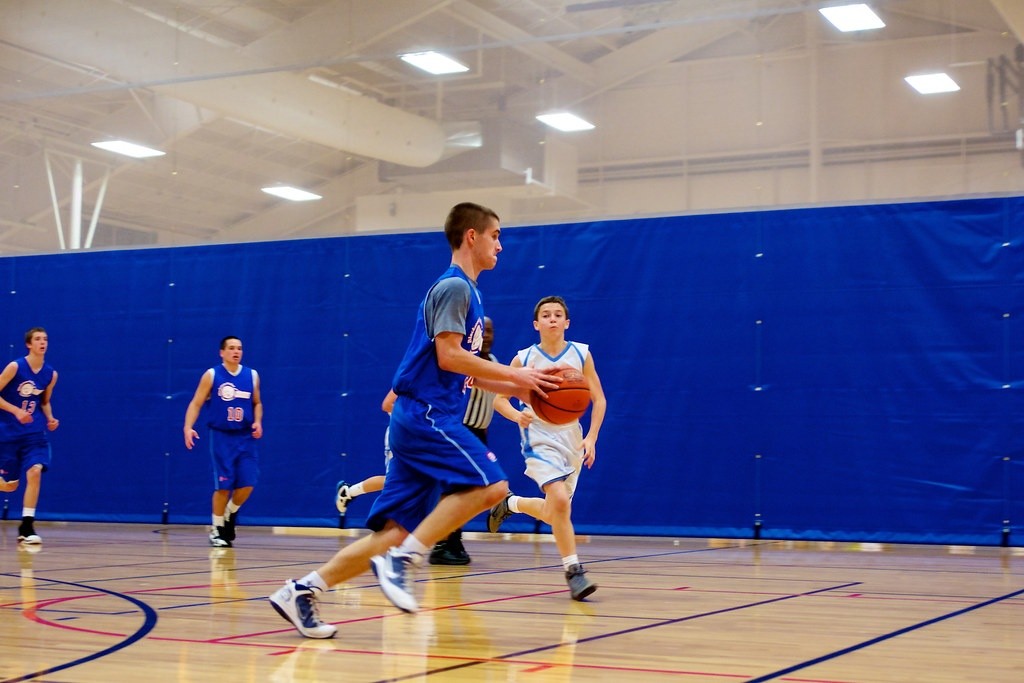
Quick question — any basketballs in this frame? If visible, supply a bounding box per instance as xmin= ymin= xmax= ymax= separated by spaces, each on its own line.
xmin=528 ymin=362 xmax=592 ymax=426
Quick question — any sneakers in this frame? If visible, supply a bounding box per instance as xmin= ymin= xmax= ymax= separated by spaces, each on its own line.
xmin=370 ymin=545 xmax=425 ymax=614
xmin=428 ymin=540 xmax=471 ymax=565
xmin=449 ymin=528 xmax=465 ymax=551
xmin=565 ymin=563 xmax=596 ymax=601
xmin=334 ymin=480 xmax=353 ymax=516
xmin=225 ymin=507 xmax=239 ymax=541
xmin=208 ymin=525 xmax=232 ymax=547
xmin=487 ymin=489 xmax=515 ymax=533
xmin=17 ymin=516 xmax=42 ymax=544
xmin=269 ymin=579 xmax=338 ymax=639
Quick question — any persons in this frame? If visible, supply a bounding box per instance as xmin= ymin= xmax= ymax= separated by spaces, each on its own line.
xmin=268 ymin=202 xmax=565 ymax=639
xmin=490 ymin=296 xmax=607 ymax=600
xmin=183 ymin=336 xmax=263 ymax=547
xmin=0 ymin=327 xmax=59 ymax=544
